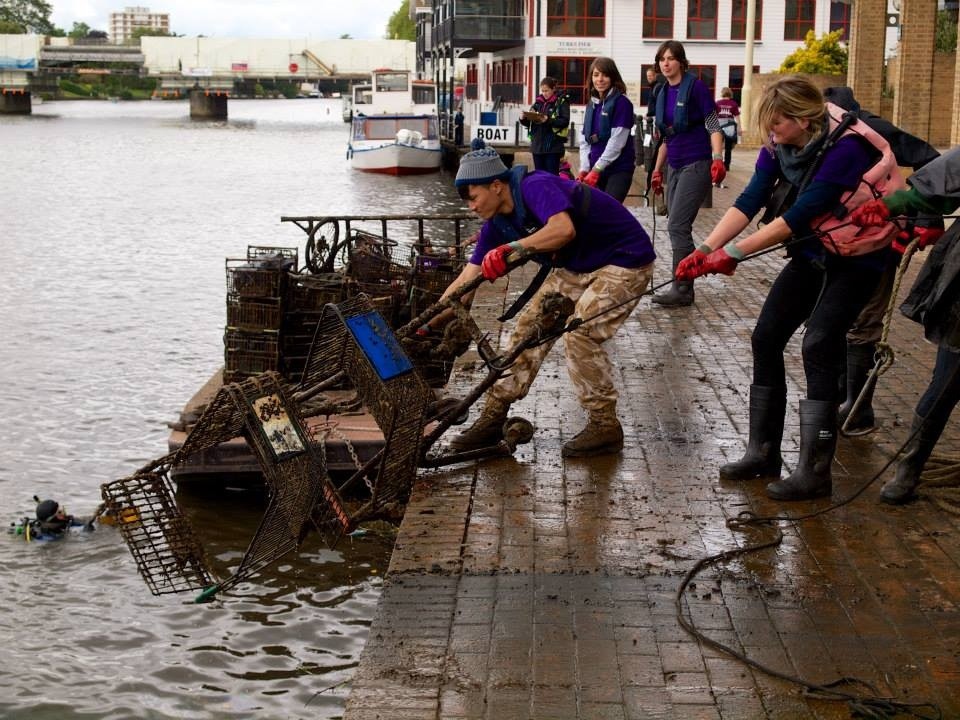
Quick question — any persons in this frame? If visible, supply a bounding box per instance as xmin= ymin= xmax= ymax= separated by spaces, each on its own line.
xmin=848 ymin=143 xmax=960 ymax=503
xmin=454 ymin=106 xmax=464 ymax=146
xmin=646 ymin=69 xmax=665 ymax=118
xmin=716 ymin=87 xmax=741 ymax=171
xmin=414 ymin=218 xmax=480 ymax=272
xmin=405 ymin=138 xmax=657 ymax=459
xmin=648 ymin=40 xmax=724 ymax=304
xmin=575 ymin=57 xmax=637 ymax=204
xmin=675 ymin=77 xmax=907 ymax=503
xmin=519 ymin=77 xmax=571 ymax=176
xmin=820 ymin=85 xmax=960 ymax=435
xmin=559 ymin=149 xmax=575 ymax=180
xmin=35 ymin=499 xmax=65 ymax=524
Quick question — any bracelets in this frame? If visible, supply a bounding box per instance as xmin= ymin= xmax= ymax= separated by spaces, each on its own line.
xmin=711 ymin=152 xmax=723 ymax=160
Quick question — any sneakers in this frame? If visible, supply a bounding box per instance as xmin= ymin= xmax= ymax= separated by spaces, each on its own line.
xmin=450 ymin=418 xmax=508 ymax=451
xmin=564 ymin=423 xmax=625 ymax=457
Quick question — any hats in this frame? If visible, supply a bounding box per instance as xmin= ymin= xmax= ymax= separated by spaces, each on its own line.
xmin=454 ymin=138 xmax=510 ymax=188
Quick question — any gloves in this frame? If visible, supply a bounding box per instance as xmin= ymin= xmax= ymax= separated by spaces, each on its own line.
xmin=675 ymin=245 xmax=712 ymax=281
xmin=576 ymin=172 xmax=587 ymax=184
xmin=416 ymin=328 xmax=425 ymax=335
xmin=912 ymin=215 xmax=946 ymax=250
xmin=709 ymin=153 xmax=727 ymax=185
xmin=481 ymin=240 xmax=522 ymax=284
xmin=849 ymin=186 xmax=931 ymax=227
xmin=583 ymin=170 xmax=600 ymax=188
xmin=701 ymin=240 xmax=745 ymax=276
xmin=650 ymin=170 xmax=664 ymax=196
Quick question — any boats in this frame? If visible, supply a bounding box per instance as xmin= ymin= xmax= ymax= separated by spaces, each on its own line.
xmin=346 ymin=68 xmax=446 ymax=176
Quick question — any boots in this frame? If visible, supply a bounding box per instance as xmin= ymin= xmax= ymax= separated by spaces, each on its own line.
xmin=651 ymin=247 xmax=694 ymax=307
xmin=838 ymin=343 xmax=879 ymax=430
xmin=765 ymin=400 xmax=839 ymax=501
xmin=880 ymin=408 xmax=949 ymax=503
xmin=720 ymin=384 xmax=787 ymax=479
